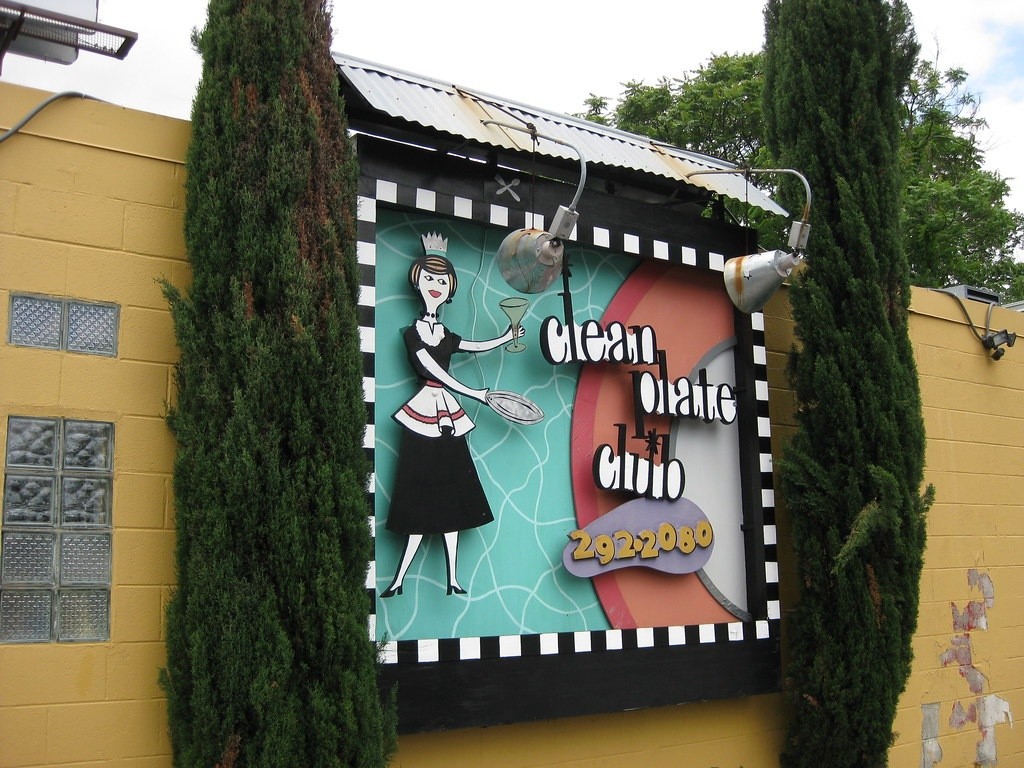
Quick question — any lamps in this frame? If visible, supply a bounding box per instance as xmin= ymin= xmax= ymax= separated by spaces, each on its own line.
xmin=685 ymin=169 xmax=812 ymax=317
xmin=982 ymin=329 xmax=1017 ymax=361
xmin=483 ymin=118 xmax=586 ymax=295
